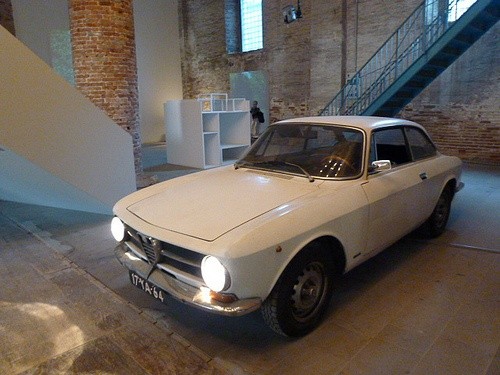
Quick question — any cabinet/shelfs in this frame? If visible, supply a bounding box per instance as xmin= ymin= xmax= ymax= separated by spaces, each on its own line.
xmin=162 ymin=92 xmax=252 ymax=170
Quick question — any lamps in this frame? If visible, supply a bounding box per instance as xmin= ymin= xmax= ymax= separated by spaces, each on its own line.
xmin=282 ymin=0 xmax=302 ymax=24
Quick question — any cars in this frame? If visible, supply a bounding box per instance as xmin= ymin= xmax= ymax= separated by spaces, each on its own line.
xmin=111 ymin=116 xmax=465 ymax=339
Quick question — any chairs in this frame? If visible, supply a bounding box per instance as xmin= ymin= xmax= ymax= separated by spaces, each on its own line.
xmin=329 ymin=140 xmax=377 ymax=168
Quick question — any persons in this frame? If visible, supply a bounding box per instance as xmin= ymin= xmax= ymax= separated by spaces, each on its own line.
xmin=250 ymin=101 xmax=261 ymax=139
